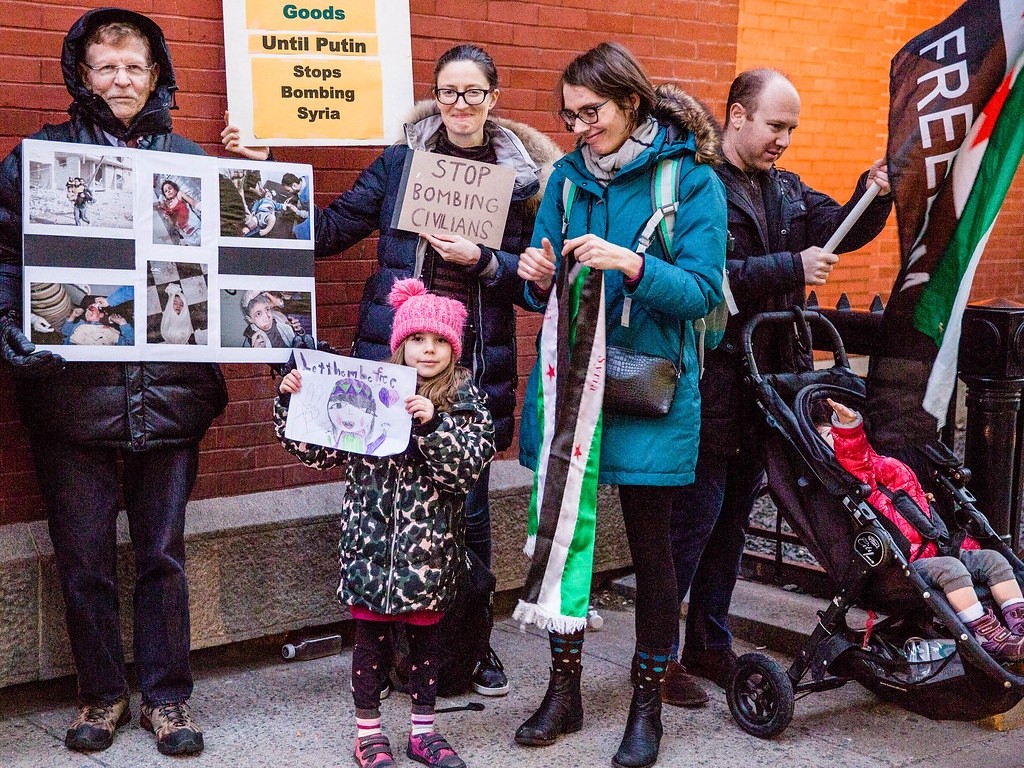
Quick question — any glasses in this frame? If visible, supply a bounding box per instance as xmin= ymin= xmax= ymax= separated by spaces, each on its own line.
xmin=433 ymin=86 xmax=493 ymax=105
xmin=559 ymin=97 xmax=614 ymax=126
xmin=83 ymin=62 xmax=154 ymax=80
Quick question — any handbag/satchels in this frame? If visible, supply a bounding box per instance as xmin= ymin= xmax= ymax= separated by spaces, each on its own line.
xmin=603 ymin=345 xmax=676 ymax=416
xmin=388 ymin=542 xmax=496 ymax=696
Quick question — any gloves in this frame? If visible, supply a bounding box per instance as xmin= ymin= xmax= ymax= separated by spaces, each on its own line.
xmin=0 ymin=310 xmax=66 ymax=379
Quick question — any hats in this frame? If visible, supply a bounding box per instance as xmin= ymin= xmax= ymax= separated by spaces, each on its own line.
xmin=388 ymin=278 xmax=467 ymax=363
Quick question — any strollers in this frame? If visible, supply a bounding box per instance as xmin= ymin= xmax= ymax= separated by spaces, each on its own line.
xmin=723 ymin=303 xmax=1024 ymax=742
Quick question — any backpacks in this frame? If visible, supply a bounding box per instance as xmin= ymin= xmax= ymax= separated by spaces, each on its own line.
xmin=562 ymin=153 xmax=738 ymax=378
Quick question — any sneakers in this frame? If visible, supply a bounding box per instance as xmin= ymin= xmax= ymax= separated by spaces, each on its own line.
xmin=65 ymin=695 xmax=131 ymax=751
xmin=680 ymin=643 xmax=739 ymax=688
xmin=963 ymin=607 xmax=1024 ymax=660
xmin=469 ymin=646 xmax=509 ymax=696
xmin=407 ymin=732 xmax=466 ymax=768
xmin=660 ymin=660 xmax=709 ymax=705
xmin=140 ymin=701 xmax=203 ymax=754
xmin=354 ymin=733 xmax=397 ymax=768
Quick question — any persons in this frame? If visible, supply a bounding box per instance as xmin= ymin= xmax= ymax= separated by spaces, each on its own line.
xmin=0 ymin=7 xmax=230 ymax=755
xmin=153 ymin=180 xmax=202 ymax=240
xmin=665 ymin=68 xmax=891 ymax=705
xmin=812 ymin=395 xmax=1024 ymax=660
xmin=221 ymin=45 xmax=566 ymax=697
xmin=272 ymin=278 xmax=496 ymax=768
xmin=241 ymin=289 xmax=311 ymax=348
xmin=30 ymin=282 xmax=134 ymax=347
xmin=515 ymin=43 xmax=728 ymax=768
xmin=66 ymin=176 xmax=95 ymax=225
xmin=173 ymin=295 xmax=184 ymax=316
xmin=243 ymin=172 xmax=311 ymax=240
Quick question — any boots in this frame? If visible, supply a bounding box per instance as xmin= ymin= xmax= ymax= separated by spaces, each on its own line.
xmin=611 ymin=693 xmax=663 ymax=768
xmin=514 ymin=665 xmax=584 ymax=745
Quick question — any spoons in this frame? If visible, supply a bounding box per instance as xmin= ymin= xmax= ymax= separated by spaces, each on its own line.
xmin=433 ymin=702 xmax=485 ymax=713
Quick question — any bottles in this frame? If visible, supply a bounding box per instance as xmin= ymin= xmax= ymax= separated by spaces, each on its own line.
xmin=587 ymin=605 xmax=604 ymax=629
xmin=281 ymin=631 xmax=342 ymax=661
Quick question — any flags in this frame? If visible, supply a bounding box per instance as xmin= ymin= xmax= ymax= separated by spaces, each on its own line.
xmin=865 ymin=0 xmax=1024 ymax=451
